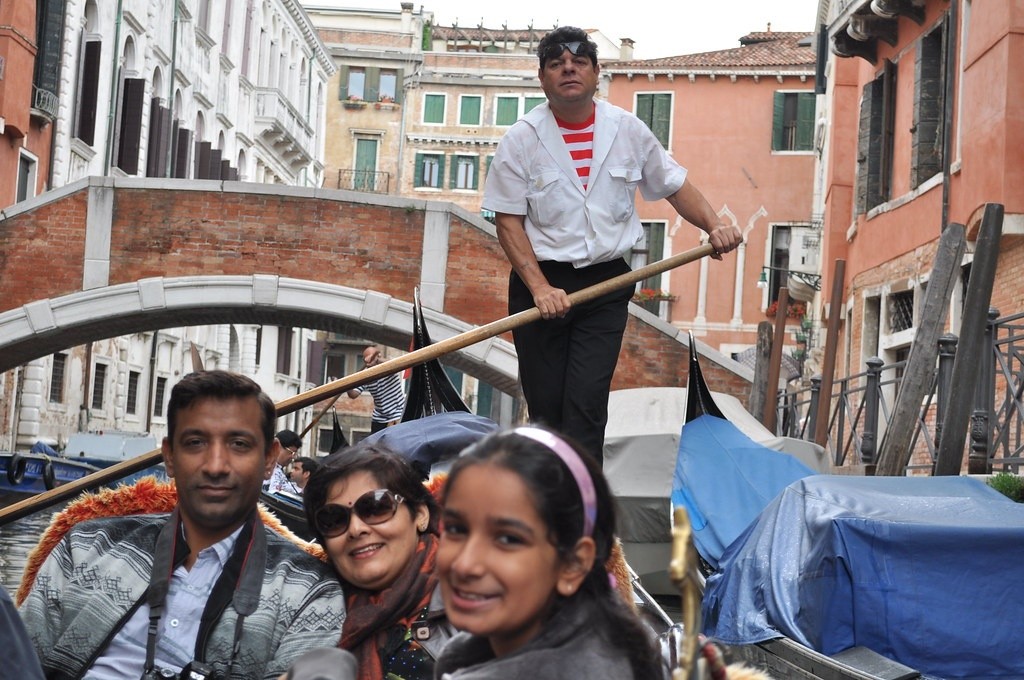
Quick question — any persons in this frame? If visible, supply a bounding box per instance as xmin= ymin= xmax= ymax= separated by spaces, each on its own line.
xmin=262 ymin=429 xmax=303 ymax=493
xmin=290 ymin=457 xmax=320 ymax=497
xmin=304 ymin=444 xmax=463 ymax=680
xmin=434 ymin=423 xmax=670 ymax=680
xmin=481 ymin=26 xmax=743 ymax=470
xmin=0 ymin=370 xmax=346 ymax=680
xmin=332 ymin=346 xmax=406 ymax=435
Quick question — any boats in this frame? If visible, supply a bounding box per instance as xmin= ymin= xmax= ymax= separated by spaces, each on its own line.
xmin=258 ymin=491 xmax=314 ymax=545
xmin=0 ymin=442 xmax=117 ymax=504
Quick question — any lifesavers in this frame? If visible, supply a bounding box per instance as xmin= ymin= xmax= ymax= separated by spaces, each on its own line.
xmin=44 ymin=460 xmax=57 ymax=491
xmin=9 ymin=451 xmax=28 ymax=487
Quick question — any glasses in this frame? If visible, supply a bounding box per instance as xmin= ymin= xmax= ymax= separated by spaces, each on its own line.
xmin=285 ymin=447 xmax=296 ymax=458
xmin=542 ymin=42 xmax=593 ymax=69
xmin=315 ymin=490 xmax=408 ymax=539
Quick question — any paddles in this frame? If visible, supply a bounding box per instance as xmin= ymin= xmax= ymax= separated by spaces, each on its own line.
xmin=1 ymin=242 xmax=718 ymax=534
